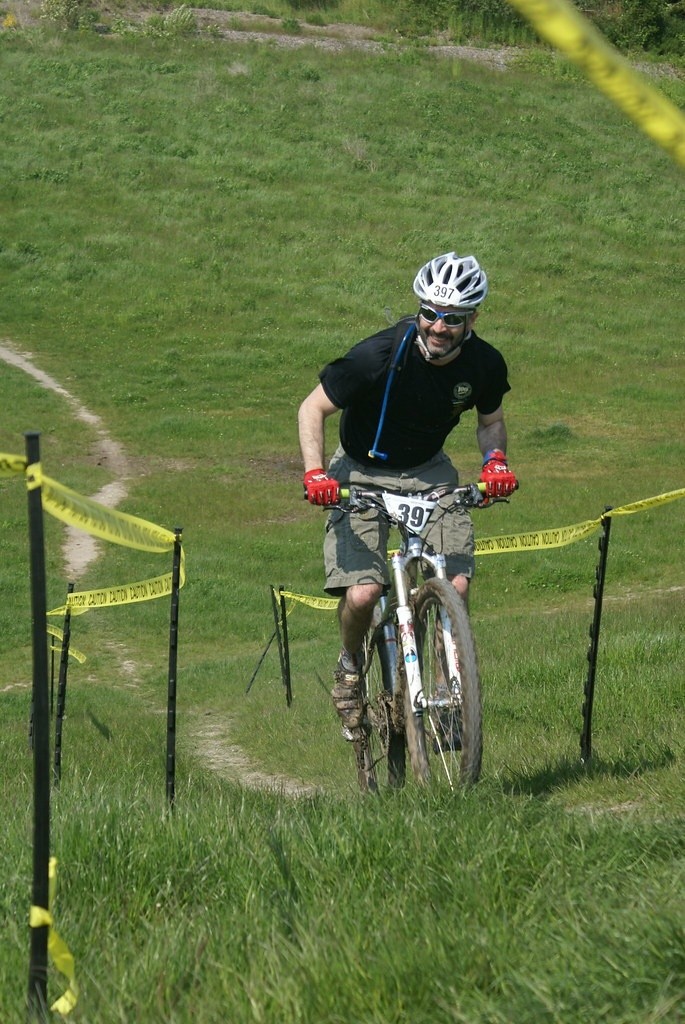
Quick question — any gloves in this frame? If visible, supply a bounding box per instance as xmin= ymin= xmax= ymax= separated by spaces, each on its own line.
xmin=478 ymin=448 xmax=516 ymax=504
xmin=303 ymin=468 xmax=340 ymax=506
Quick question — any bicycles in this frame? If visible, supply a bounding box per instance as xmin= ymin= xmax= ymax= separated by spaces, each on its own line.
xmin=304 ymin=477 xmax=521 ymax=798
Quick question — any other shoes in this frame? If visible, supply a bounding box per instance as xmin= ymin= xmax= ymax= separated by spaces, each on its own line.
xmin=438 ymin=707 xmax=464 ymax=744
xmin=333 ymin=646 xmax=365 ymax=728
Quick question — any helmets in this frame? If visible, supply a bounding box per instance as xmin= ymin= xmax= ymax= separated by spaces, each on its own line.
xmin=412 ymin=251 xmax=488 ymax=308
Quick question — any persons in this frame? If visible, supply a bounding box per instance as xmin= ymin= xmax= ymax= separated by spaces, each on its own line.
xmin=298 ymin=252 xmax=517 ymax=750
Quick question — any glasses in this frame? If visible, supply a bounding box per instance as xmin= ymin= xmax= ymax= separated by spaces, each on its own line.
xmin=420 ymin=303 xmax=473 ymax=326
